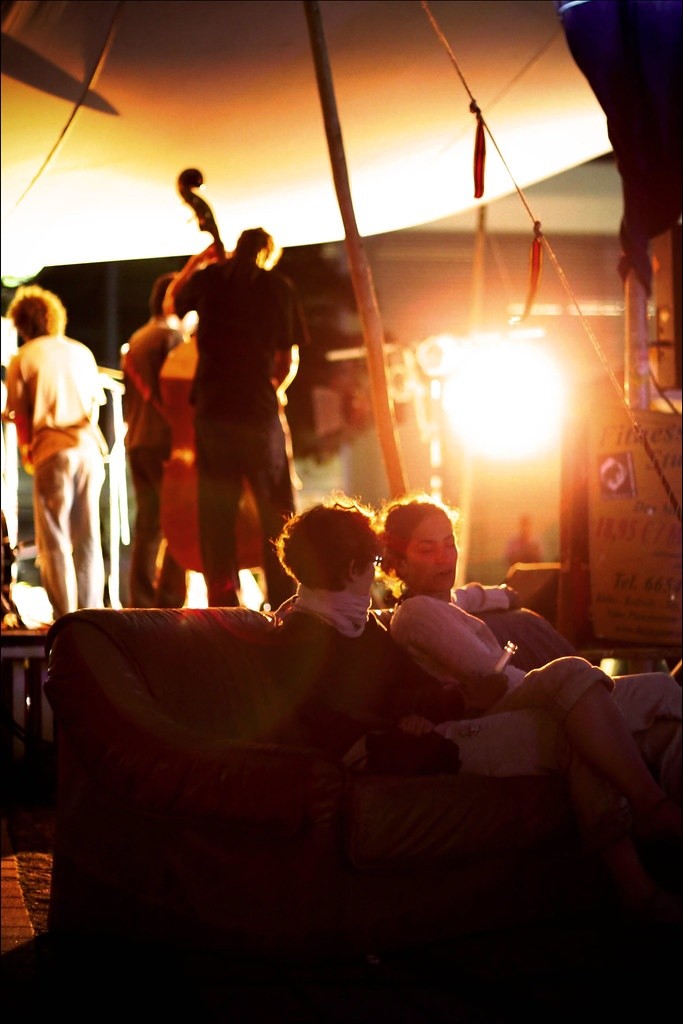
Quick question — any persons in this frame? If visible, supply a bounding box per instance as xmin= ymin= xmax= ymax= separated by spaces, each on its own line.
xmin=5 ymin=285 xmax=106 ymax=623
xmin=163 ymin=229 xmax=310 ymax=612
xmin=275 ymin=503 xmax=683 ymax=928
xmin=121 ymin=273 xmax=198 ymax=608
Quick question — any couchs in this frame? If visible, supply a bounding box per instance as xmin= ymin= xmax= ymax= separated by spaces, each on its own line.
xmin=43 ymin=607 xmax=683 ymax=979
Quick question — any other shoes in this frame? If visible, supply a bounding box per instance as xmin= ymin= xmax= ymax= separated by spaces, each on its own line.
xmin=615 ymin=887 xmax=683 ymax=924
xmin=630 ymin=795 xmax=683 ymax=840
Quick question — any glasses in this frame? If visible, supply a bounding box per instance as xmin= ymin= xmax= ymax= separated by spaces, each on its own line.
xmin=368 ymin=556 xmax=383 ymax=566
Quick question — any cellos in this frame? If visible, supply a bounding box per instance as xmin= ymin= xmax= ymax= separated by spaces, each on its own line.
xmin=156 ymin=168 xmax=305 ymax=571
